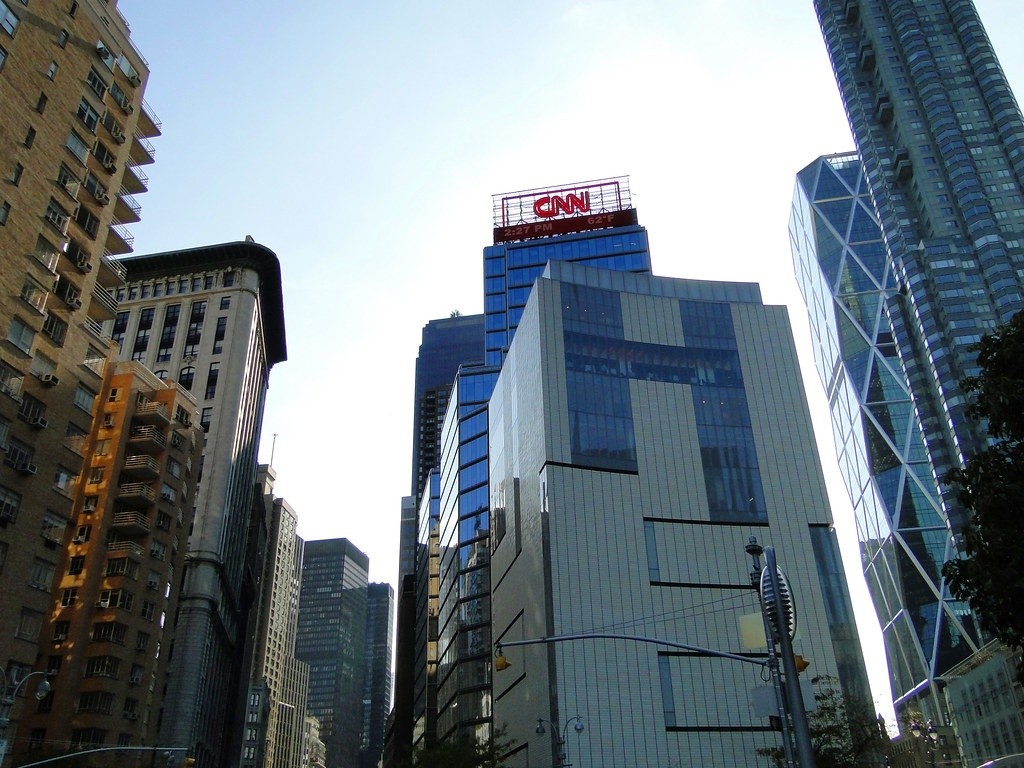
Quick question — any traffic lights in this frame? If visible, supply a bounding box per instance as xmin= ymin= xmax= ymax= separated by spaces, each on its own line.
xmin=184 ymin=757 xmax=196 ymax=768
xmin=792 ymin=652 xmax=810 ymax=674
xmin=494 ymin=655 xmax=513 ymax=673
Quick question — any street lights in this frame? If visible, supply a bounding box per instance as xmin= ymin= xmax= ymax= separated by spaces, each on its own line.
xmin=0 ymin=665 xmax=51 ymax=768
xmin=535 ymin=713 xmax=586 ymax=768
